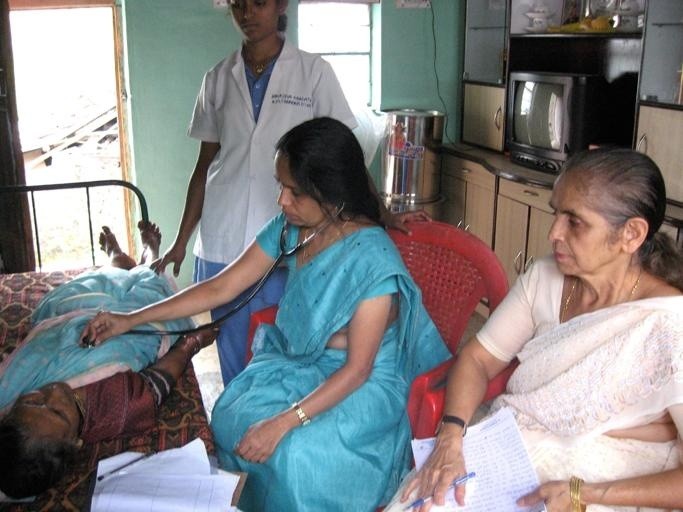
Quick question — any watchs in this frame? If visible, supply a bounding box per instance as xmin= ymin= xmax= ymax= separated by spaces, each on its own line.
xmin=288 ymin=401 xmax=311 ymax=430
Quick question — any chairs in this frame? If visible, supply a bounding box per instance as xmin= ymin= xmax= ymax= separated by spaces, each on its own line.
xmin=413 ymin=356 xmax=520 ymax=438
xmin=245 ymin=221 xmax=511 ymax=439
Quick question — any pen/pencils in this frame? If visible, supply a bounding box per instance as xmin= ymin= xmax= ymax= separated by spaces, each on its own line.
xmin=97 ymin=448 xmax=162 ymax=482
xmin=403 ymin=473 xmax=477 ymax=510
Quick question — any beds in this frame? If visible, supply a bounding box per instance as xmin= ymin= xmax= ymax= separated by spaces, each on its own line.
xmin=0 ymin=180 xmax=224 ymax=512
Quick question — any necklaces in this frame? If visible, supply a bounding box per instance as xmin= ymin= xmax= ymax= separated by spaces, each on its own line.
xmin=241 ymin=46 xmax=283 ymax=75
xmin=300 ymin=224 xmax=350 ymax=262
xmin=383 ymin=142 xmax=683 ymax=512
xmin=557 ymin=269 xmax=645 ymax=325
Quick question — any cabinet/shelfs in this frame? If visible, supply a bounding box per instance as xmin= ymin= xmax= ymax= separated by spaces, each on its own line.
xmin=440 ymin=141 xmax=683 ymax=321
xmin=459 ymin=0 xmax=683 ymax=210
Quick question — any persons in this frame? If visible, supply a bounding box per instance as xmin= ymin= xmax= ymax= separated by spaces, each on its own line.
xmin=74 ymin=114 xmax=453 ymax=510
xmin=148 ymin=1 xmax=364 ymax=392
xmin=0 ymin=219 xmax=222 ymax=501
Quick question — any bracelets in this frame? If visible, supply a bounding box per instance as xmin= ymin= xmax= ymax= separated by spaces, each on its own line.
xmin=439 ymin=411 xmax=468 ymax=439
xmin=567 ymin=475 xmax=587 ymax=512
xmin=179 ymin=333 xmax=203 ymax=352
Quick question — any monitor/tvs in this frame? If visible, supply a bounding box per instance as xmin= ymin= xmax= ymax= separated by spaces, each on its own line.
xmin=508 ymin=70 xmax=601 ymax=176
